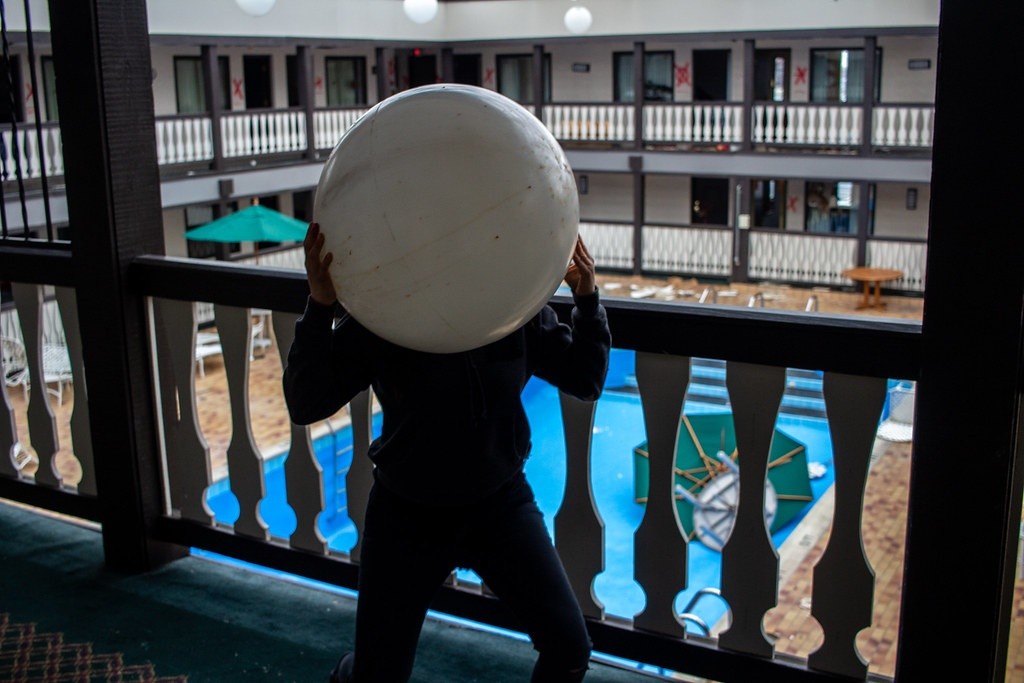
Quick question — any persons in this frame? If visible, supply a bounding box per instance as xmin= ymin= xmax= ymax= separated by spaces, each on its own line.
xmin=282 ymin=218 xmax=612 ymax=682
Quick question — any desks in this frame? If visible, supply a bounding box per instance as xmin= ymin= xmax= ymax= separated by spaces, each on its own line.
xmin=675 ymin=450 xmax=776 ymax=553
xmin=842 ymin=268 xmax=903 ymax=310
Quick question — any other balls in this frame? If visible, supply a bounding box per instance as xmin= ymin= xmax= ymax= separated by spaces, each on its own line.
xmin=311 ymin=82 xmax=582 ymax=355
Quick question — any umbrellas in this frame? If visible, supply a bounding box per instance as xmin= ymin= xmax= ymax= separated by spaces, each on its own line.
xmin=180 ymin=197 xmax=309 ymax=265
xmin=633 ymin=410 xmax=814 ymax=547
